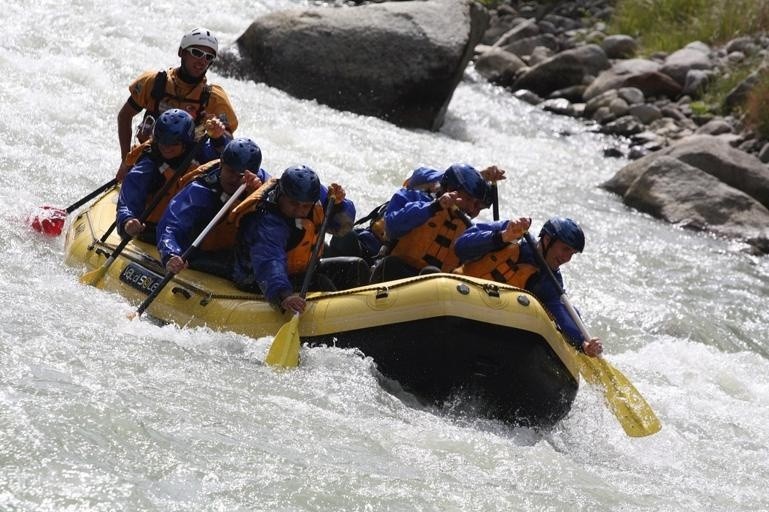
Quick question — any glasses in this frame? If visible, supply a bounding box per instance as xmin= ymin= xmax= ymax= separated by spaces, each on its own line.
xmin=188 ymin=47 xmax=216 ymax=63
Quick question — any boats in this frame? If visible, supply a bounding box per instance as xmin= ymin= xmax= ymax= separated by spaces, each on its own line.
xmin=63 ymin=178 xmax=578 ymax=434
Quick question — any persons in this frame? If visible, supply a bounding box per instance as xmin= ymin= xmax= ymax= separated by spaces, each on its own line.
xmin=364 ymin=165 xmax=506 ymax=242
xmin=157 ymin=138 xmax=270 ymax=280
xmin=371 ymin=161 xmax=488 ymax=285
xmin=115 ymin=28 xmax=239 ymax=183
xmin=232 ymin=165 xmax=370 ymax=315
xmin=454 ymin=218 xmax=604 ymax=358
xmin=117 ymin=109 xmax=228 ymax=247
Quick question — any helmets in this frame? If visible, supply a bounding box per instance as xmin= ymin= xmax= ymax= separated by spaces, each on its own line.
xmin=180 ymin=27 xmax=218 ymax=57
xmin=540 ymin=215 xmax=585 ymax=253
xmin=154 ymin=108 xmax=195 ymax=145
xmin=279 ymin=163 xmax=320 ymax=202
xmin=443 ymin=162 xmax=487 ymax=199
xmin=222 ymin=138 xmax=262 ymax=174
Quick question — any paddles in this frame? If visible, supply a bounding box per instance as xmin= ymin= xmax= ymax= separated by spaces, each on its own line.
xmin=80 ymin=133 xmax=211 ymax=290
xmin=523 ymin=230 xmax=662 ymax=437
xmin=263 ymin=194 xmax=337 ymax=368
xmin=31 ymin=120 xmax=205 ymax=234
